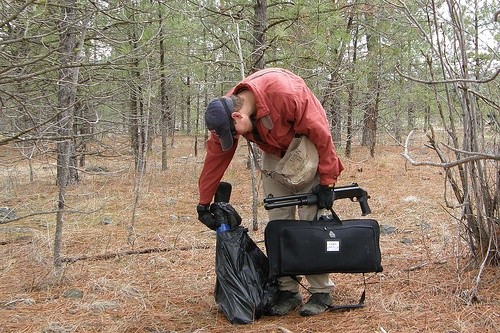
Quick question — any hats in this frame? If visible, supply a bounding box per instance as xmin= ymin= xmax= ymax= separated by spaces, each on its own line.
xmin=204 ymin=97 xmax=234 ymax=151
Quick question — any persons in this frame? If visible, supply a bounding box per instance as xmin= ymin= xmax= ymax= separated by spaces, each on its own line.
xmin=197 ymin=68 xmax=345 ymax=316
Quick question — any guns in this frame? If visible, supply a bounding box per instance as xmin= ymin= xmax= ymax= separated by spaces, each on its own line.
xmin=263 ymin=181 xmax=372 ymax=216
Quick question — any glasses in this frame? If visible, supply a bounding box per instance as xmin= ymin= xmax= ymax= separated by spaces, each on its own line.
xmin=219 ymin=97 xmax=237 ymax=136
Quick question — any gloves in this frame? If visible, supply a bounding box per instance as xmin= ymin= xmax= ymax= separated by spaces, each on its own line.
xmin=197 ymin=204 xmax=217 ymax=231
xmin=312 ymin=184 xmax=335 ymax=210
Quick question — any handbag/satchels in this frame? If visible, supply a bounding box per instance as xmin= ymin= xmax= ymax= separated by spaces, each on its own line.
xmin=265 ymin=203 xmax=384 ymax=278
xmin=268 ymin=135 xmax=319 ymax=193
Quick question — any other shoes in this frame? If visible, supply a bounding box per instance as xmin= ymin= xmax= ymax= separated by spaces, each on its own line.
xmin=266 ymin=291 xmax=302 ymax=316
xmin=300 ymin=293 xmax=333 ymax=316
